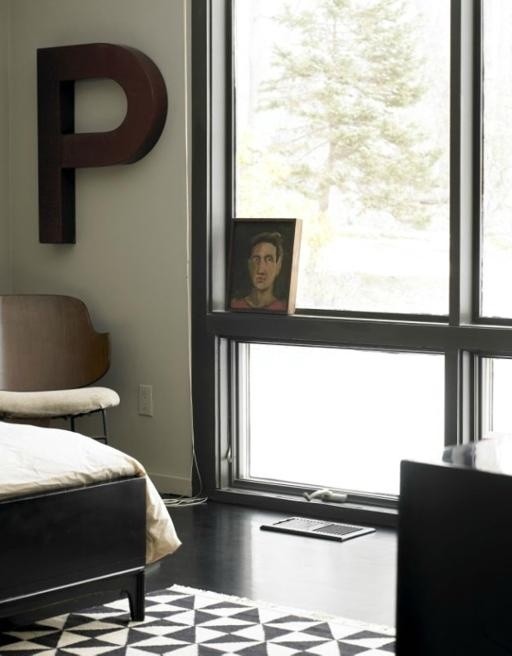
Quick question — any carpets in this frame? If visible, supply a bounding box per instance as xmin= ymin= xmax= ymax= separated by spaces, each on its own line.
xmin=0 ymin=580 xmax=397 ymax=656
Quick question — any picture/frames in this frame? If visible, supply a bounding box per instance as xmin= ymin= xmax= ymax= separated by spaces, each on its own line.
xmin=221 ymin=214 xmax=303 ymax=315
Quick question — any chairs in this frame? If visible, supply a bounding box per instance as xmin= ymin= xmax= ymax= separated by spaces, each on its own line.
xmin=0 ymin=292 xmax=122 ymax=445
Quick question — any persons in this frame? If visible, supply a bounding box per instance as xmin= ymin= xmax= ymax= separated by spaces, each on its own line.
xmin=228 ymin=230 xmax=287 ymax=313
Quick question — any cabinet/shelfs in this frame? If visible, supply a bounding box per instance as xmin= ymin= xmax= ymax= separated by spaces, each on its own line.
xmin=397 ymin=437 xmax=512 ymax=655
